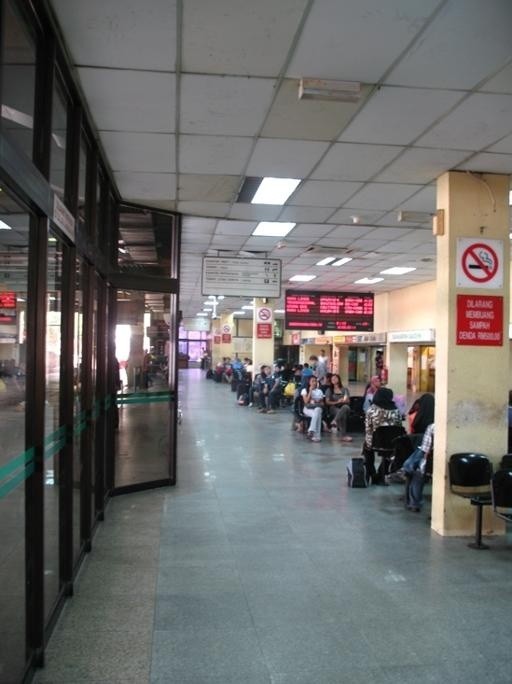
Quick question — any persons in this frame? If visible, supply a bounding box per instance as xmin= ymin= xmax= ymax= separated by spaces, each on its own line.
xmin=362 ymin=377 xmax=434 ymax=512
xmin=375 ymin=351 xmax=383 ymax=376
xmin=201 ymin=347 xmax=354 ymax=442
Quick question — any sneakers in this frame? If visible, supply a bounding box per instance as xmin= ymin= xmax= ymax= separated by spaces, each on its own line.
xmin=407 ymin=507 xmax=419 ymax=512
xmin=384 ymin=467 xmax=408 ymax=485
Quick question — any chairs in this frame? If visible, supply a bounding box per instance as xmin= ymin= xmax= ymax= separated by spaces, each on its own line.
xmin=363 ymin=425 xmax=425 ymax=507
xmin=449 ymin=453 xmax=512 ymax=550
xmin=254 ymin=373 xmax=341 ymax=407
xmin=209 ymin=367 xmax=251 ymax=398
xmin=293 ymin=396 xmax=366 ymax=435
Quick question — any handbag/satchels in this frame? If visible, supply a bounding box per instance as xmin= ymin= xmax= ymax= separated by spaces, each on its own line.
xmin=237 ymin=393 xmax=249 ymax=406
xmin=344 ymin=457 xmax=373 ymax=488
